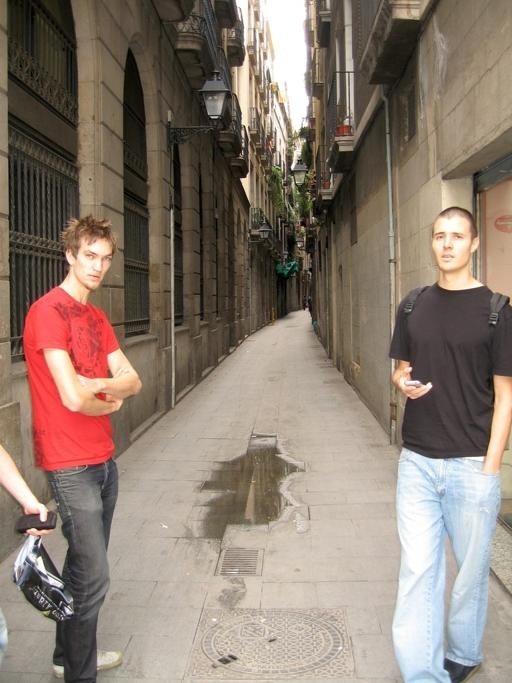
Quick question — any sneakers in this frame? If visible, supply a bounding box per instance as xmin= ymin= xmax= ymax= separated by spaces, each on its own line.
xmin=53 ymin=650 xmax=123 ymax=677
xmin=444 ymin=657 xmax=481 ymax=682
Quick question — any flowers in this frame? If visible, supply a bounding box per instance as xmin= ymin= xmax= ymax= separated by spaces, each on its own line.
xmin=335 ymin=111 xmax=353 ymax=125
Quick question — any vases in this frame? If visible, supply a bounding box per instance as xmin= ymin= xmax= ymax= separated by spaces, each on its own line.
xmin=322 ymin=180 xmax=330 ymax=188
xmin=336 ymin=124 xmax=353 ymax=136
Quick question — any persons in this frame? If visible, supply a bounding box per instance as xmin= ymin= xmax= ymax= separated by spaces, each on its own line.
xmin=385 ymin=205 xmax=511 ymax=682
xmin=0 ymin=443 xmax=55 ymax=665
xmin=22 ymin=214 xmax=145 ymax=681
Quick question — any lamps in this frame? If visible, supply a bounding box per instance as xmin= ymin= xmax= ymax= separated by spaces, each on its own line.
xmin=249 ymin=222 xmax=272 ymax=247
xmin=292 ymin=158 xmax=318 ymax=196
xmin=168 ymin=68 xmax=234 ymax=145
xmin=295 ymin=237 xmax=307 ymax=252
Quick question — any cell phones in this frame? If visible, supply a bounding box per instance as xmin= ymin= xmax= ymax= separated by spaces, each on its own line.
xmin=15 ymin=510 xmax=57 ymax=533
xmin=405 ymin=380 xmax=425 ymax=388
